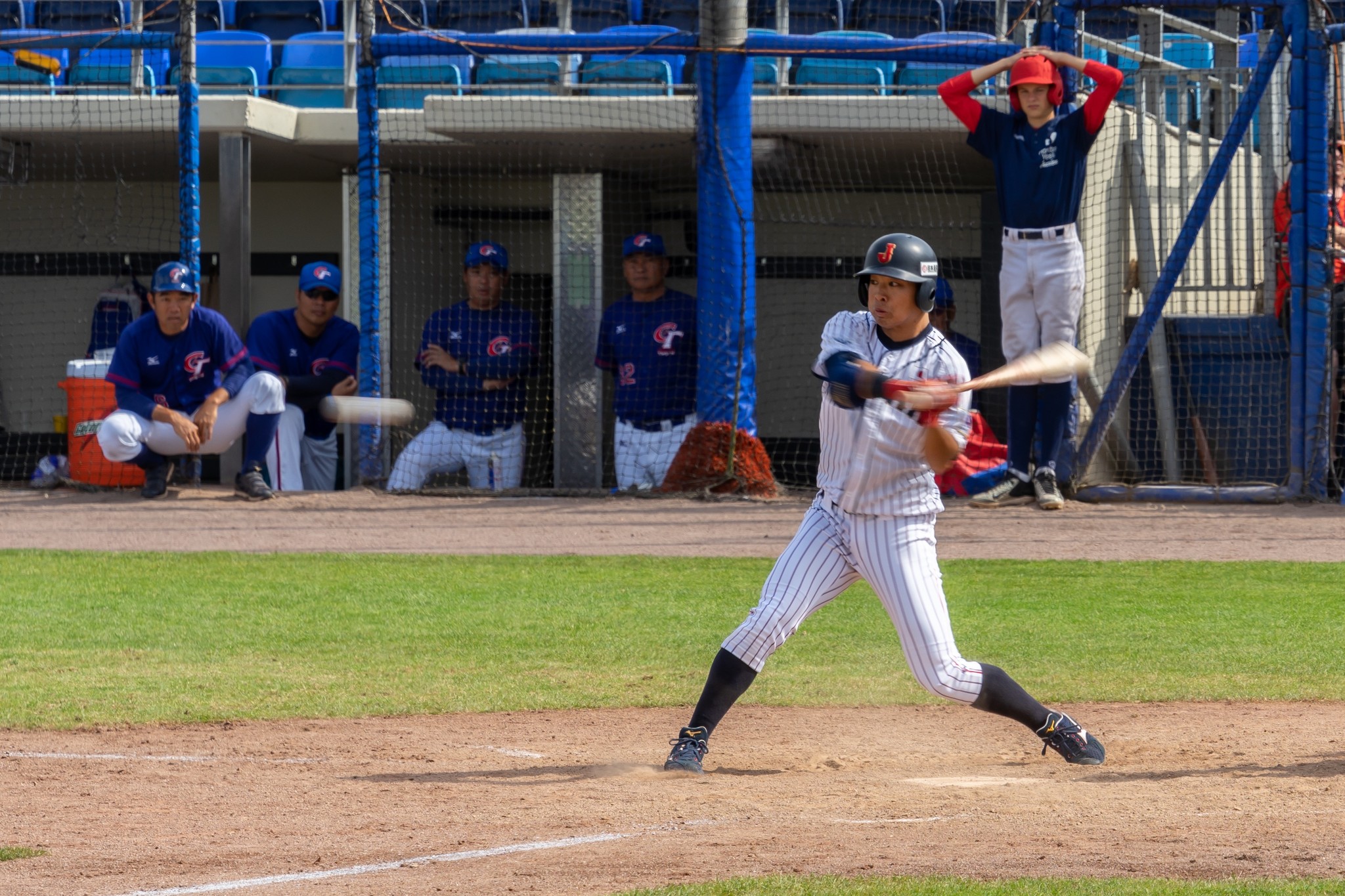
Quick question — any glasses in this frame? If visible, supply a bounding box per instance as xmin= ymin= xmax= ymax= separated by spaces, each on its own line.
xmin=303 ymin=288 xmax=338 ymax=301
xmin=1326 ymin=145 xmax=1345 ymax=155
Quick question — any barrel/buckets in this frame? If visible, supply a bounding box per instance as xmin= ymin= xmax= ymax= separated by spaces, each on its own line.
xmin=57 ymin=359 xmax=146 ymax=487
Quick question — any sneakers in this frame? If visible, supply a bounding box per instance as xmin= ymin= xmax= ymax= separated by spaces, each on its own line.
xmin=970 ymin=471 xmax=1036 ymax=510
xmin=1030 ymin=470 xmax=1064 ymax=510
xmin=1038 ymin=708 xmax=1106 ymax=767
xmin=234 ymin=457 xmax=275 ymax=501
xmin=663 ymin=725 xmax=710 ymax=772
xmin=144 ymin=459 xmax=176 ymax=500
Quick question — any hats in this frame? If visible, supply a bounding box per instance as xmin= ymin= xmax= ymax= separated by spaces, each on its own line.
xmin=151 ymin=262 xmax=196 ymax=294
xmin=621 ymin=231 xmax=666 ymax=258
xmin=464 ymin=242 xmax=511 ymax=275
xmin=298 ymin=262 xmax=343 ymax=294
xmin=1285 ymin=126 xmax=1345 ymax=156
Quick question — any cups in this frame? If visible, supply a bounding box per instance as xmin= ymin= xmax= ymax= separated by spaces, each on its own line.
xmin=54 ymin=416 xmax=68 ymax=433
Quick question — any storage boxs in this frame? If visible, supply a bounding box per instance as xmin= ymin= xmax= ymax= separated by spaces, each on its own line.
xmin=58 ymin=377 xmax=148 ymax=486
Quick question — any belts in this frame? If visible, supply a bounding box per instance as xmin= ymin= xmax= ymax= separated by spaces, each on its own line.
xmin=618 ymin=418 xmax=687 ymax=434
xmin=455 ymin=424 xmax=512 ymax=436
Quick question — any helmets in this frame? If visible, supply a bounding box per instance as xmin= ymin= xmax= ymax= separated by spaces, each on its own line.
xmin=858 ymin=232 xmax=939 ymax=315
xmin=1007 ymin=53 xmax=1064 ymax=111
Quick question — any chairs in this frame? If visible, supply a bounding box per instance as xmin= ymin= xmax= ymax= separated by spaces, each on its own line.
xmin=0 ymin=0 xmax=1263 ymax=153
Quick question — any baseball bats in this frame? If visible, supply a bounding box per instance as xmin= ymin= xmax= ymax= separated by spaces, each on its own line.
xmin=1172 ymin=340 xmax=1218 ymax=487
xmin=890 ymin=340 xmax=1093 ymax=412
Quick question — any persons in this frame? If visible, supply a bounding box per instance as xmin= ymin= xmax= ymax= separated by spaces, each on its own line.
xmin=1274 ymin=125 xmax=1345 ymax=363
xmin=592 ymin=234 xmax=697 ymax=491
xmin=247 ymin=262 xmax=360 ymax=492
xmin=659 ymin=230 xmax=1104 ymax=778
xmin=383 ymin=240 xmax=537 ymax=491
xmin=96 ymin=261 xmax=287 ymax=503
xmin=938 ymin=44 xmax=1123 ymax=510
